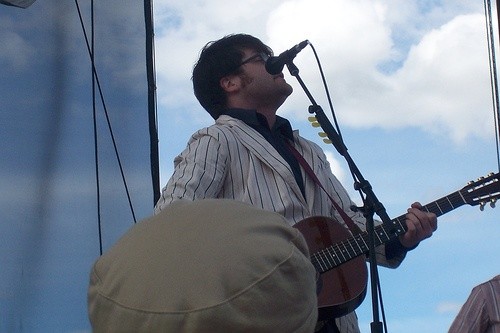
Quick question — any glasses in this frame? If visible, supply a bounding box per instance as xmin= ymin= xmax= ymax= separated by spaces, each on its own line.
xmin=240 ymin=52 xmax=269 ymax=67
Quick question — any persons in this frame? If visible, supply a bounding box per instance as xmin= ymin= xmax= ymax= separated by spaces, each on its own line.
xmin=448 ymin=275 xmax=500 ymax=333
xmin=152 ymin=33 xmax=437 ymax=333
xmin=88 ymin=197 xmax=318 ymax=333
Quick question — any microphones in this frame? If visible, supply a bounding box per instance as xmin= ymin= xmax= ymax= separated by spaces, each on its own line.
xmin=265 ymin=39 xmax=308 ymax=75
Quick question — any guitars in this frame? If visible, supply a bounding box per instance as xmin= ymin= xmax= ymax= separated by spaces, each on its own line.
xmin=292 ymin=172 xmax=500 ymax=319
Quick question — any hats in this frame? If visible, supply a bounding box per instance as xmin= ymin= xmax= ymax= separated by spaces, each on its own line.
xmin=88 ymin=198 xmax=317 ymax=333
